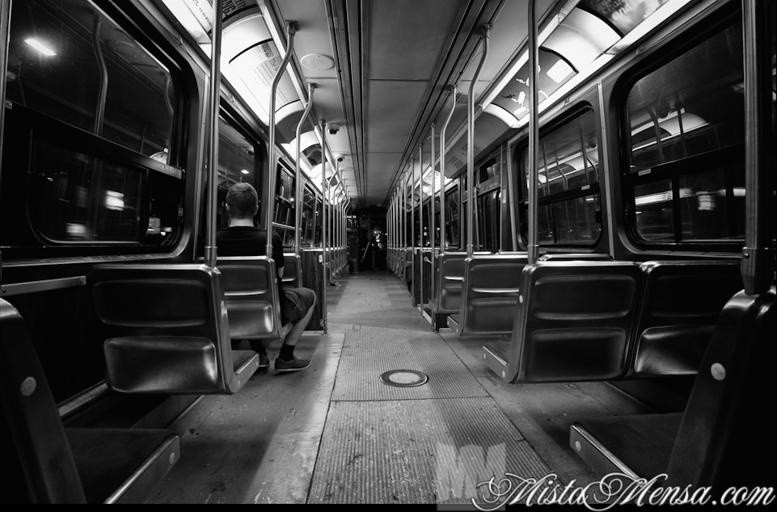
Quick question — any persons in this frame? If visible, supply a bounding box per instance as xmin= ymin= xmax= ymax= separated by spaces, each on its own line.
xmin=216 ymin=182 xmax=316 ymax=372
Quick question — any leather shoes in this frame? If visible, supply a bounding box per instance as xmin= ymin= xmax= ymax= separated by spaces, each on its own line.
xmin=258 ymin=354 xmax=269 ymax=367
xmin=274 ymin=357 xmax=311 ymax=371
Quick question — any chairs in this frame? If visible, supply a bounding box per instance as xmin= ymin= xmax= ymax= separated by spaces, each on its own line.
xmin=205 ymin=245 xmax=349 ymax=339
xmin=0 ymin=295 xmax=182 ymax=504
xmin=83 ymin=260 xmax=261 ymax=395
xmin=571 ymin=286 xmax=777 ymax=503
